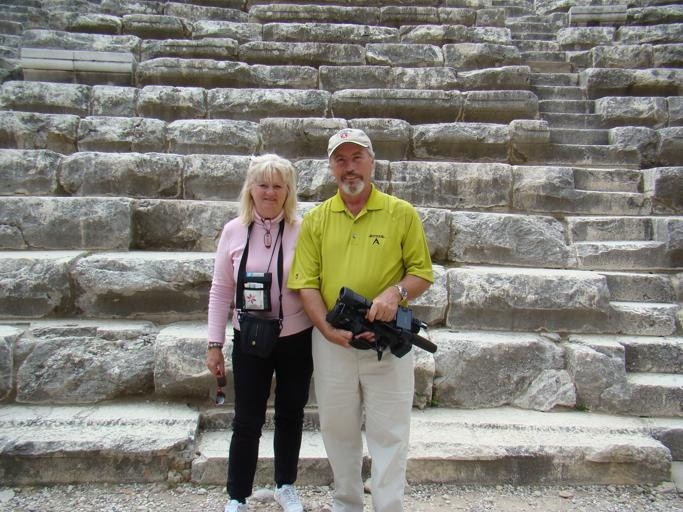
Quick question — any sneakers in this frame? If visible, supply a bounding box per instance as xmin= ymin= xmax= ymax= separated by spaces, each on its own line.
xmin=272 ymin=482 xmax=303 ymax=511
xmin=223 ymin=498 xmax=248 ymax=512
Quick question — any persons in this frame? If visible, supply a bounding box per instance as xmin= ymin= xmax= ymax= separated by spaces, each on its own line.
xmin=202 ymin=152 xmax=313 ymax=512
xmin=285 ymin=127 xmax=436 ymax=512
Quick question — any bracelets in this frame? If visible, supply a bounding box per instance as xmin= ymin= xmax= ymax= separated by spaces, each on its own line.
xmin=205 ymin=342 xmax=225 ymax=350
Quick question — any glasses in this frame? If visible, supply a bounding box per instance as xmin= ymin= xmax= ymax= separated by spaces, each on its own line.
xmin=213 ymin=371 xmax=226 ymax=407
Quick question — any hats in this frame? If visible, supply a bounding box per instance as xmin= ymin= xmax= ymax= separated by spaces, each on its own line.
xmin=325 ymin=127 xmax=374 ymax=160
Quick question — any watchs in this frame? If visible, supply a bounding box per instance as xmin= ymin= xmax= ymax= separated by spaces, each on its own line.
xmin=393 ymin=283 xmax=408 ymax=302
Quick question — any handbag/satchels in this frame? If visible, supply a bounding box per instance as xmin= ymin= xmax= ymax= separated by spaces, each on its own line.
xmin=237 ymin=270 xmax=273 ymax=312
xmin=237 ymin=311 xmax=281 ymax=360
